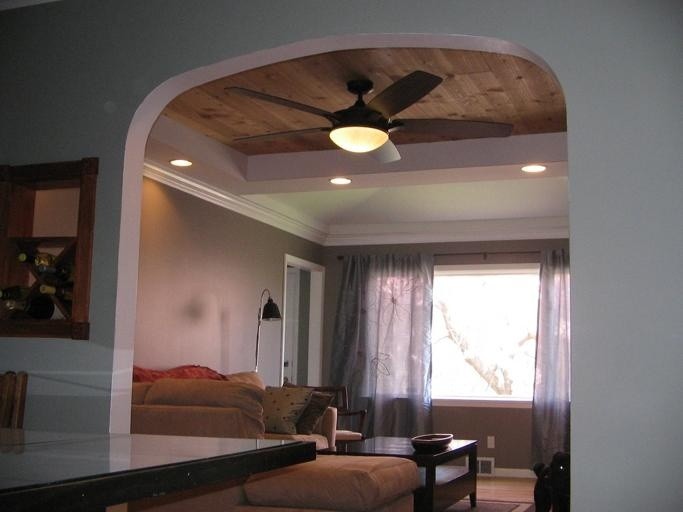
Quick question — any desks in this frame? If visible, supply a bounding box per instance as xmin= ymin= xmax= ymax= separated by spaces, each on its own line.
xmin=0 ymin=429 xmax=317 ymax=512
xmin=318 ymin=436 xmax=480 ymax=512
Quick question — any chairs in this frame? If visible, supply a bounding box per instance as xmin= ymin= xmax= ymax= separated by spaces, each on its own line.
xmin=0 ymin=369 xmax=28 ymax=430
xmin=287 ymin=379 xmax=368 ymax=453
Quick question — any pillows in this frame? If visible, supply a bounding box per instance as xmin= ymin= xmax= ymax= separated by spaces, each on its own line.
xmin=261 ymin=386 xmax=316 ymax=436
xmin=281 ymin=381 xmax=335 ymax=436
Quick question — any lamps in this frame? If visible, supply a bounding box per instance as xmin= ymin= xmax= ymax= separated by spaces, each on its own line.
xmin=327 ymin=124 xmax=391 ymax=154
xmin=253 ymin=288 xmax=281 ymax=374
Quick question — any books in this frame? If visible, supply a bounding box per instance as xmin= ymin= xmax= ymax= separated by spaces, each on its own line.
xmin=0 ymin=428 xmax=26 ymax=455
xmin=0 ymin=370 xmax=29 ymax=429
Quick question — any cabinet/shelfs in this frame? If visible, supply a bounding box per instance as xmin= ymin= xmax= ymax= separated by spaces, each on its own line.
xmin=0 ymin=235 xmax=77 ymax=322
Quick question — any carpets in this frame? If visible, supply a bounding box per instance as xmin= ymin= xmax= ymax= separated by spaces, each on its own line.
xmin=444 ymin=496 xmax=535 ymax=512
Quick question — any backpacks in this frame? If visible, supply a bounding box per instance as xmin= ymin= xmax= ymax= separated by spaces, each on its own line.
xmin=534 ymin=453 xmax=570 ymax=512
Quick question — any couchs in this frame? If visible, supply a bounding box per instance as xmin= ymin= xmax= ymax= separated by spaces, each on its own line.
xmin=125 ymin=372 xmax=422 ymax=512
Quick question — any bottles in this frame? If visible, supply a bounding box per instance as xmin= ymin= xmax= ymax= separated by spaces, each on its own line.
xmin=0 ymin=253 xmax=75 ymax=318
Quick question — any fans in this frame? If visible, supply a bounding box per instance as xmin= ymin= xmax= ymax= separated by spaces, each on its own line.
xmin=222 ymin=69 xmax=514 ymax=165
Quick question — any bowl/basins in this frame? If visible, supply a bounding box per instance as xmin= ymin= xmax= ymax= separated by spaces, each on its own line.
xmin=410 ymin=432 xmax=453 ymax=451
